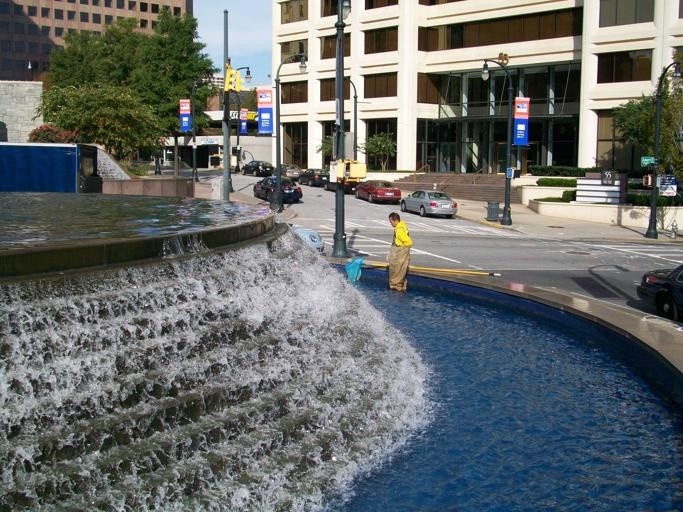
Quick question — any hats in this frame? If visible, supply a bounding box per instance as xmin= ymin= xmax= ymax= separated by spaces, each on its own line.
xmin=240 ymin=158 xmax=359 ymax=204
xmin=353 ymin=180 xmax=401 ymax=204
xmin=398 ymin=189 xmax=457 ymax=219
xmin=289 ymin=224 xmax=327 ymax=256
xmin=636 ymin=259 xmax=682 ymax=325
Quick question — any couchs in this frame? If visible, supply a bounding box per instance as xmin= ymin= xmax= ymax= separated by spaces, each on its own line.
xmin=233 ymin=72 xmax=244 ymax=93
xmin=641 ymin=174 xmax=651 ymax=187
xmin=223 ymin=70 xmax=234 ymax=93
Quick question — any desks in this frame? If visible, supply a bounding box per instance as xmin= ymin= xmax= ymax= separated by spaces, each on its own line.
xmin=644 ymin=60 xmax=682 ymax=240
xmin=332 ymin=1 xmax=353 ymax=262
xmin=274 ymin=52 xmax=308 ymax=211
xmin=481 ymin=58 xmax=512 ymax=225
xmin=190 ymin=78 xmax=212 ymax=182
xmin=229 ymin=66 xmax=251 ymax=194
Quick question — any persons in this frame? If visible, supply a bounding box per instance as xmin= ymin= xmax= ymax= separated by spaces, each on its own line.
xmin=385 ymin=212 xmax=413 ymax=293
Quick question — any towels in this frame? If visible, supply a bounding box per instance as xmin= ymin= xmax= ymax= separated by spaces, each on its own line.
xmin=487 ymin=201 xmax=499 ymax=222
